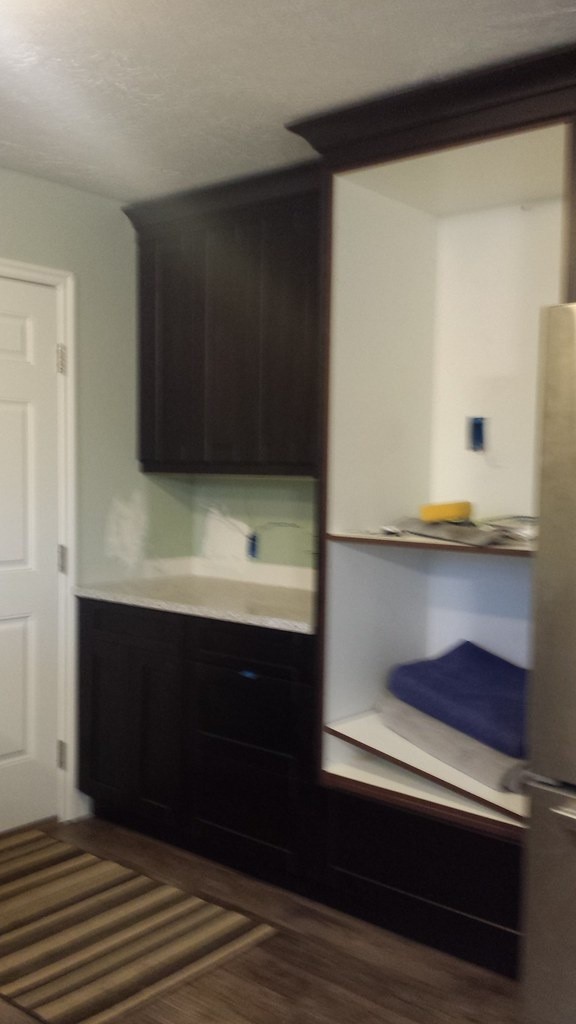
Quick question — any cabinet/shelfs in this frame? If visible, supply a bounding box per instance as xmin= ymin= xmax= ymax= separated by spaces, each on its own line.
xmin=119 ymin=153 xmax=325 ymax=477
xmin=184 ymin=614 xmax=313 ymax=896
xmin=74 ymin=596 xmax=195 ymax=851
xmin=282 ymin=38 xmax=574 ymax=978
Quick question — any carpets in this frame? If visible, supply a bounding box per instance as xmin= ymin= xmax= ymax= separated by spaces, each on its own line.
xmin=0 ymin=828 xmax=282 ymax=1024
